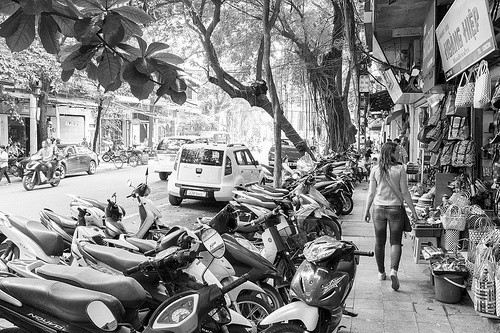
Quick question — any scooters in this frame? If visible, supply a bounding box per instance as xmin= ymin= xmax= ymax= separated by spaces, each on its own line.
xmin=0 ymin=120 xmax=373 ymax=333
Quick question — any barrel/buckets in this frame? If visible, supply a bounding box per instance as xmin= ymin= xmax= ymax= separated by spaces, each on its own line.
xmin=432 ymin=272 xmax=466 ymax=303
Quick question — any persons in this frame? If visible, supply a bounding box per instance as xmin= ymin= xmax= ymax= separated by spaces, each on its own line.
xmin=0 ymin=133 xmax=57 ymax=181
xmin=363 ymin=142 xmax=419 ymax=291
xmin=362 ymin=136 xmax=408 ymax=165
xmin=81 ymin=138 xmax=88 ymax=147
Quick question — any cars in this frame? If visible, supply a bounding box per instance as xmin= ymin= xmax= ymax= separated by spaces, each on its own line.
xmin=268 ymin=139 xmax=319 ymax=169
xmin=16 ymin=144 xmax=100 ymax=179
xmin=167 ymin=143 xmax=266 ymax=205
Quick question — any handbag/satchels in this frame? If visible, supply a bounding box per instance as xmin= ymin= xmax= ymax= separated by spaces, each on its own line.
xmin=445 ymin=58 xmax=500 ymax=117
xmin=418 ymin=96 xmax=448 ymax=169
xmin=402 ymin=206 xmax=413 ymax=232
xmin=438 ymin=185 xmax=500 ymax=317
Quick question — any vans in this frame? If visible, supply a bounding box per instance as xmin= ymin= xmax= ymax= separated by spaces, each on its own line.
xmin=153 ymin=136 xmax=214 ymax=180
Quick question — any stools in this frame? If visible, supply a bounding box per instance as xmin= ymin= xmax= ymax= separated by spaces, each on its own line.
xmin=412 ymin=237 xmax=437 ymax=263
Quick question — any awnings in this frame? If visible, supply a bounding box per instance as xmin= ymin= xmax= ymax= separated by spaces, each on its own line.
xmin=370 ymin=90 xmax=395 ymax=111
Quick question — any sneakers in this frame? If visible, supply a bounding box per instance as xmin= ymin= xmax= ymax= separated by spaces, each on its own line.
xmin=378 ymin=272 xmax=386 ymax=281
xmin=390 ymin=268 xmax=399 ymax=290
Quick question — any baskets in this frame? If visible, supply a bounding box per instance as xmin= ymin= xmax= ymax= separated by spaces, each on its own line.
xmin=440 ymin=205 xmax=466 ymax=231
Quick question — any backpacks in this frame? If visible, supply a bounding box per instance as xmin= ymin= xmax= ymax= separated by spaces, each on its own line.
xmin=440 ymin=116 xmax=476 ymax=167
